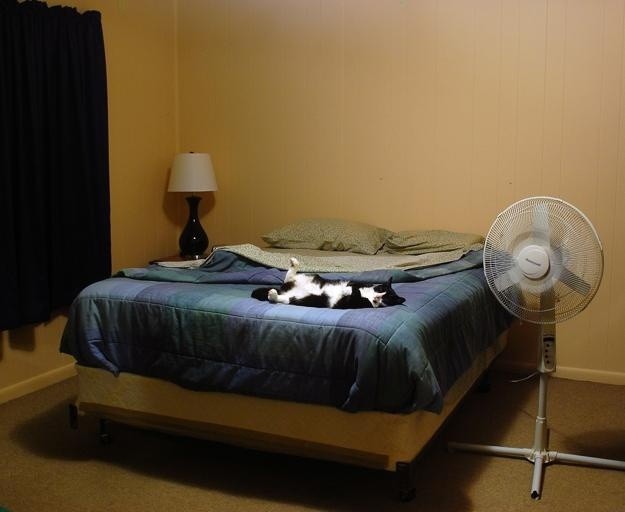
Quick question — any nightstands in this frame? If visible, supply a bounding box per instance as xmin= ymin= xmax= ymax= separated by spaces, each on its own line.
xmin=147 ymin=253 xmax=209 ymax=267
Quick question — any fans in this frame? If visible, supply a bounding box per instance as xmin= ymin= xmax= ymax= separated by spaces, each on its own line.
xmin=443 ymin=190 xmax=625 ymax=503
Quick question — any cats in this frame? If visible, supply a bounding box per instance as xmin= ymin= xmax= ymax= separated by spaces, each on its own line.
xmin=252 ymin=257 xmax=406 ymax=309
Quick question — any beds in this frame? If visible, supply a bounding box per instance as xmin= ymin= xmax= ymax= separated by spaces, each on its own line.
xmin=59 ymin=216 xmax=526 ymax=502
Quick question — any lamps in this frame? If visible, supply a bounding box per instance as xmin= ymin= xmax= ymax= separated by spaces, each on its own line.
xmin=166 ymin=146 xmax=220 ymax=262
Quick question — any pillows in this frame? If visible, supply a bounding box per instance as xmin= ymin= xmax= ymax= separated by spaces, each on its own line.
xmin=386 ymin=230 xmax=486 ymax=255
xmin=255 ymin=216 xmax=391 ymax=254
